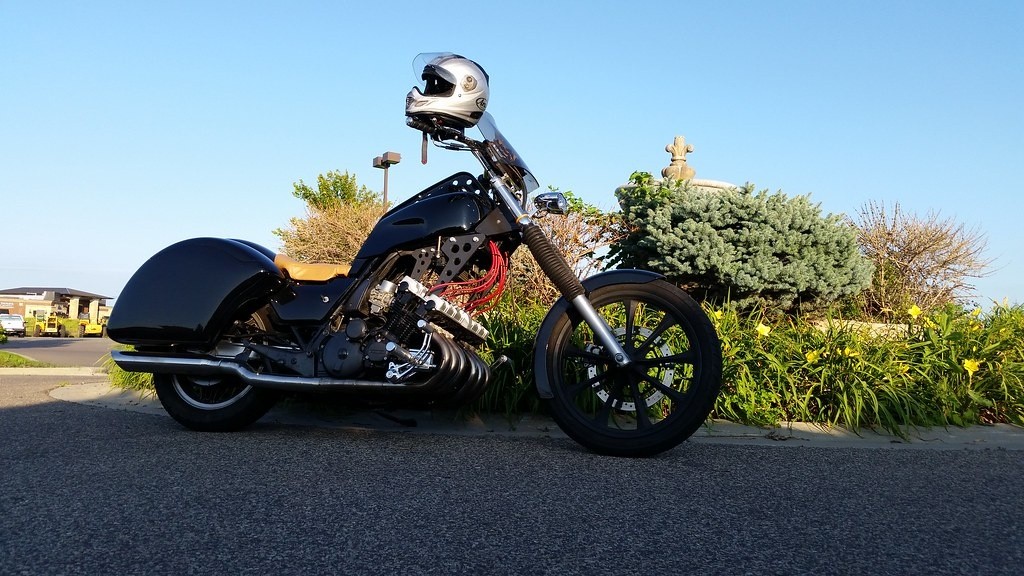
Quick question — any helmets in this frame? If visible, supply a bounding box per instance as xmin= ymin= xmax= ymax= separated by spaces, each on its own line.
xmin=405 ymin=54 xmax=490 ymax=127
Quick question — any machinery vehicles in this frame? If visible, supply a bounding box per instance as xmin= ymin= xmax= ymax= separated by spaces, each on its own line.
xmin=24 ymin=309 xmax=105 ymax=338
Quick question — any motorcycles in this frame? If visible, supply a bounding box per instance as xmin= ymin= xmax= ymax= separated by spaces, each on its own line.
xmin=103 ymin=111 xmax=725 ymax=456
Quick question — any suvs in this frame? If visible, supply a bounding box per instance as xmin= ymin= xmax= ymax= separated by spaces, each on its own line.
xmin=0 ymin=314 xmax=29 ymax=337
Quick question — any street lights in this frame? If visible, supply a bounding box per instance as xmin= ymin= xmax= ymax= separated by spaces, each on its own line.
xmin=372 ymin=150 xmax=401 ymax=216
